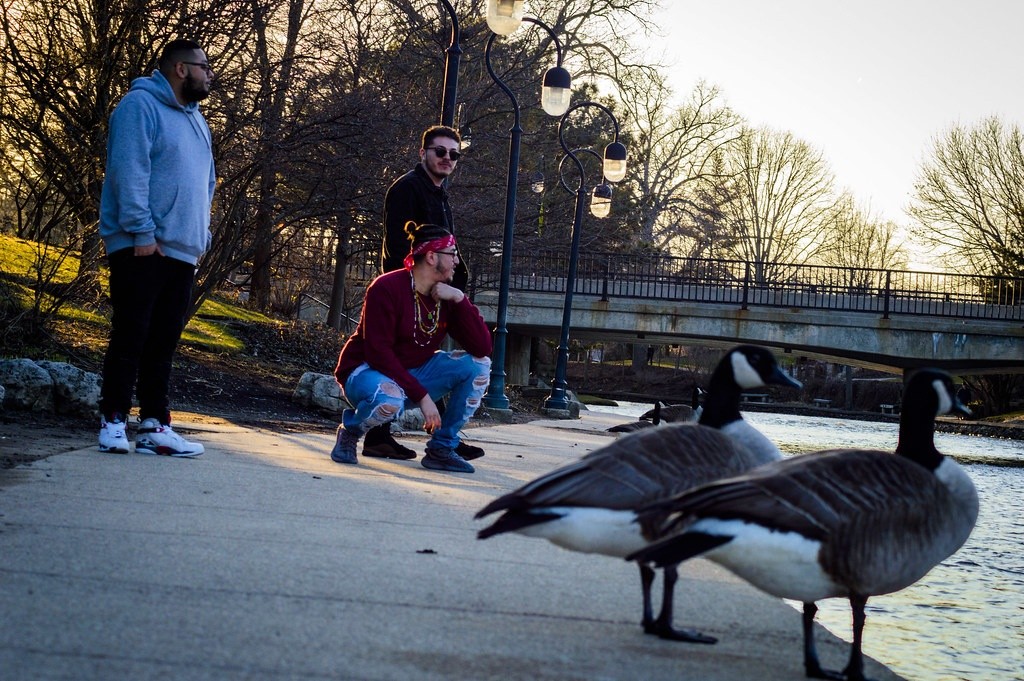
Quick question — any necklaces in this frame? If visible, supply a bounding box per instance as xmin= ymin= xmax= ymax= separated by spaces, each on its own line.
xmin=408 ymin=267 xmax=442 ymax=349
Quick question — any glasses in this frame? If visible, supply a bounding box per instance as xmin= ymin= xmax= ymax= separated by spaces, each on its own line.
xmin=436 ymin=250 xmax=458 ymax=256
xmin=425 ymin=147 xmax=461 ymax=160
xmin=183 ymin=60 xmax=211 ymax=69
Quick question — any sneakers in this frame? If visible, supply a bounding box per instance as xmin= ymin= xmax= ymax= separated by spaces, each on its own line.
xmin=422 ymin=447 xmax=475 ymax=472
xmin=134 ymin=419 xmax=204 ymax=458
xmin=98 ymin=414 xmax=131 ymax=453
xmin=454 ymin=442 xmax=484 ymax=460
xmin=331 ymin=423 xmax=359 ymax=463
xmin=363 ymin=431 xmax=416 ymax=460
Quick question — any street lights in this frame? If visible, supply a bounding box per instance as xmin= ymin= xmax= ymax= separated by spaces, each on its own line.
xmin=433 ymin=0 xmax=523 ymax=181
xmin=543 ymin=101 xmax=628 ymax=419
xmin=480 ymin=15 xmax=571 ymax=410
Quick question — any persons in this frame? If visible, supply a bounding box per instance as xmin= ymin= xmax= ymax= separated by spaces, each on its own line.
xmin=359 ymin=123 xmax=486 ymax=462
xmin=331 ymin=221 xmax=496 ymax=476
xmin=94 ymin=40 xmax=214 ymax=459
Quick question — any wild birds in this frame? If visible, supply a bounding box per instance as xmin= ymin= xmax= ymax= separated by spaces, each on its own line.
xmin=471 ymin=342 xmax=805 ymax=645
xmin=621 ymin=367 xmax=981 ymax=680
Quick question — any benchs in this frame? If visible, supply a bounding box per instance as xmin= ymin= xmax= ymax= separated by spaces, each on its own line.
xmin=812 ymin=398 xmax=832 ymax=409
xmin=879 ymin=404 xmax=900 ymax=415
xmin=740 ymin=393 xmax=770 ymax=403
